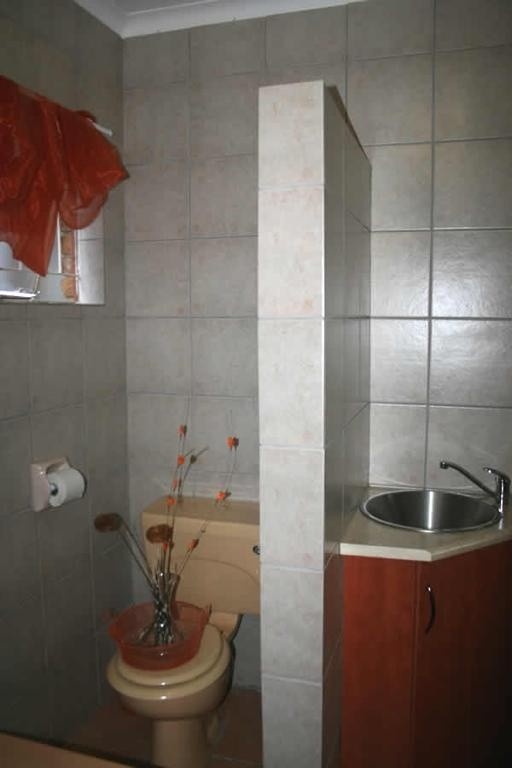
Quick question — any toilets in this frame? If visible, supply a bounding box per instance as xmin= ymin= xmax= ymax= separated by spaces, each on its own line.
xmin=104 ymin=495 xmax=260 ymax=767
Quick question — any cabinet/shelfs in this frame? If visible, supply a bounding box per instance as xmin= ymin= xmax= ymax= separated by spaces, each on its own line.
xmin=340 ymin=538 xmax=511 ymax=767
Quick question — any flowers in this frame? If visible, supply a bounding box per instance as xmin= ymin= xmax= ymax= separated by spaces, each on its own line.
xmin=109 ymin=421 xmax=236 ymax=642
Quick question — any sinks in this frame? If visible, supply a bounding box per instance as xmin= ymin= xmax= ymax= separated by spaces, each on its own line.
xmin=360 ymin=488 xmax=501 ymax=533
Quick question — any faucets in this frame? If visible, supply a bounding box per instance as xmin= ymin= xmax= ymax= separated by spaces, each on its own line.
xmin=438 ymin=460 xmax=509 ymax=507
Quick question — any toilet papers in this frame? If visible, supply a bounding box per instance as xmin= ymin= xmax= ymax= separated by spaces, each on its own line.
xmin=47 ymin=468 xmax=86 ymax=507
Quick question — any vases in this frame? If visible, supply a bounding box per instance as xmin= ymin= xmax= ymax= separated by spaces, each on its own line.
xmin=134 ymin=573 xmax=185 ymax=649
